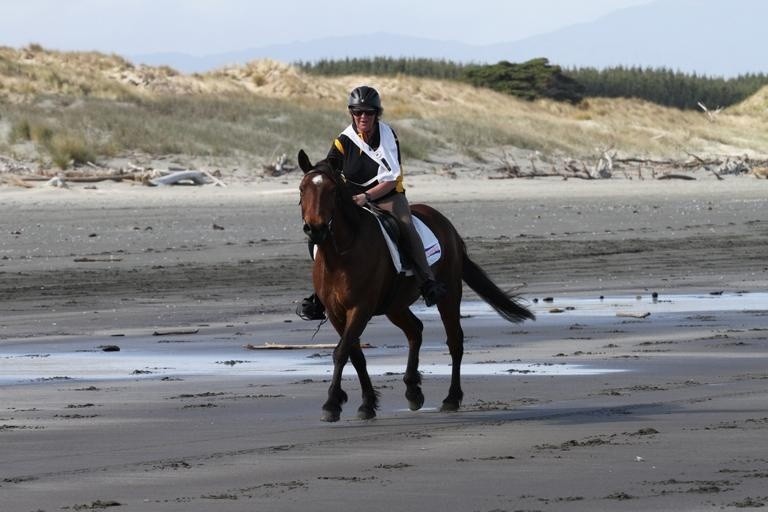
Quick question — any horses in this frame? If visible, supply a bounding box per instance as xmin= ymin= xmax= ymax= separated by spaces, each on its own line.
xmin=294 ymin=147 xmax=537 ymax=424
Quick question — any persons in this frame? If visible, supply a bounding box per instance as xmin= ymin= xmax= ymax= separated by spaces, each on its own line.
xmin=296 ymin=86 xmax=445 ymax=320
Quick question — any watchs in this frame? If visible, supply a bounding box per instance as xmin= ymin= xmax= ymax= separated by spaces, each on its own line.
xmin=364 ymin=192 xmax=371 ymax=202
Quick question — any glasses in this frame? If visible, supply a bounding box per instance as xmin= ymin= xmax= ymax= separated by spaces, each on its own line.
xmin=350 ymin=107 xmax=378 ymax=117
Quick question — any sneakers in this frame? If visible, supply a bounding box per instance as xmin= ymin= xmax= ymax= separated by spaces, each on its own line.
xmin=422 ymin=280 xmax=447 ymax=303
xmin=301 ymin=294 xmax=326 ymax=318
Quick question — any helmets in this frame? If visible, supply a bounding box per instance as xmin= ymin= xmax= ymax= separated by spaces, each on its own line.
xmin=347 ymin=86 xmax=384 ymax=117
